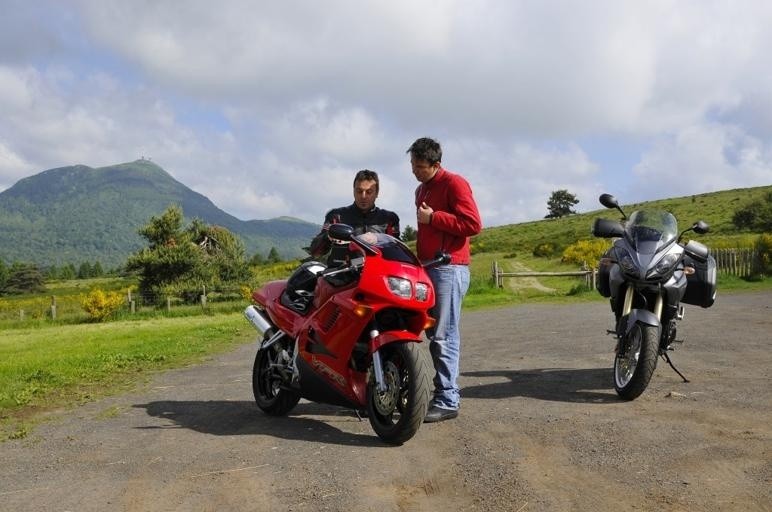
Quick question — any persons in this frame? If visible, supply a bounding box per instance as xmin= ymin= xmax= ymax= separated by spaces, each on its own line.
xmin=308 ymin=168 xmax=400 ymax=267
xmin=406 ymin=136 xmax=483 ymax=424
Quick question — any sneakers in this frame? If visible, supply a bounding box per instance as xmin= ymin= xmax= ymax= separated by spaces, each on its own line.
xmin=424 ymin=399 xmax=458 ymax=422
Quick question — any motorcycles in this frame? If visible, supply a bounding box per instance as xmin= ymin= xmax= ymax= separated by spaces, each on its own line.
xmin=244 ymin=220 xmax=452 ymax=446
xmin=593 ymin=193 xmax=717 ymax=401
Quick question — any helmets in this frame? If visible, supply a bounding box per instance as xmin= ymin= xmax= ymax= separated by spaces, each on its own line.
xmin=286 ymin=260 xmax=326 ymax=309
